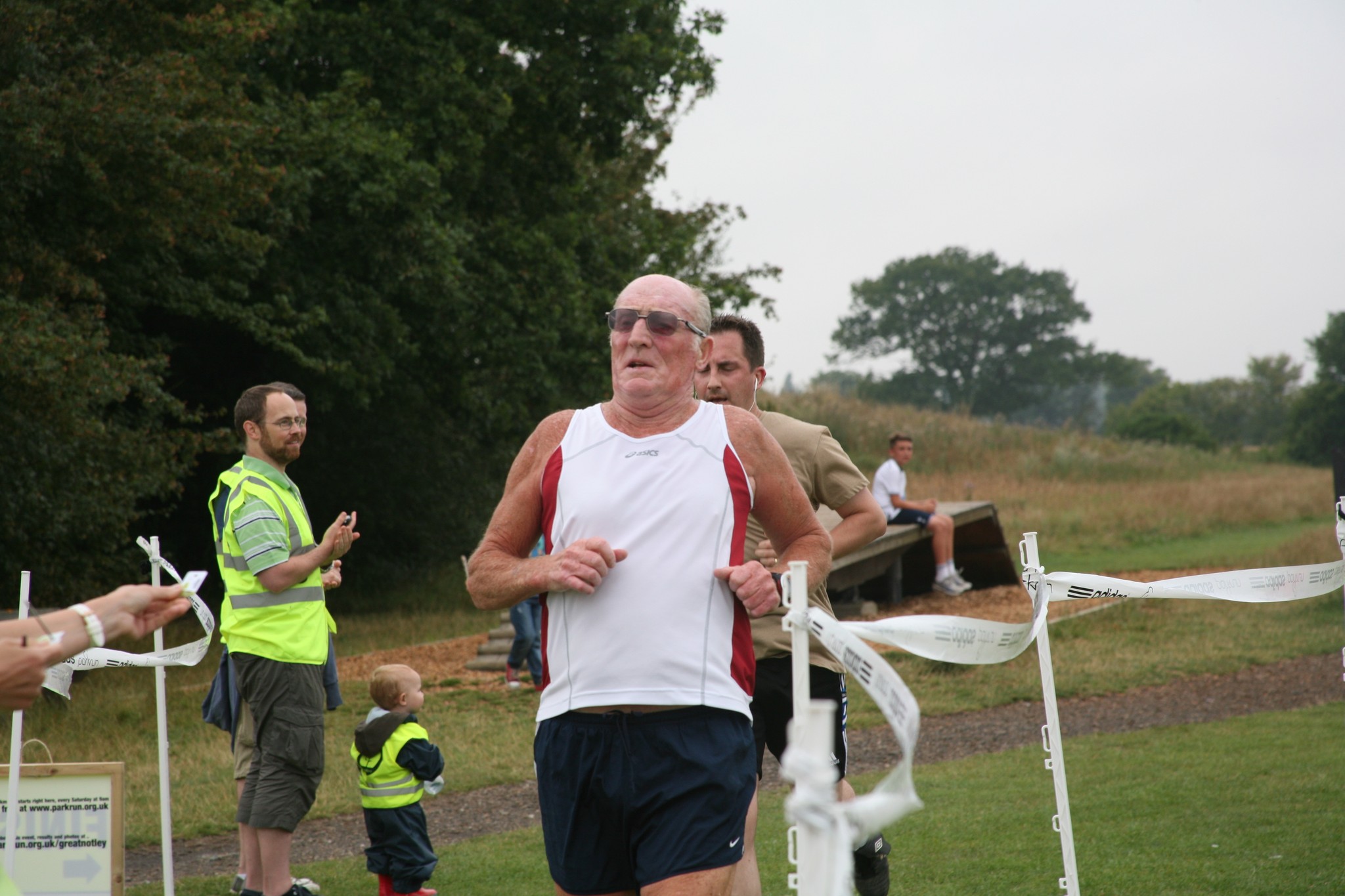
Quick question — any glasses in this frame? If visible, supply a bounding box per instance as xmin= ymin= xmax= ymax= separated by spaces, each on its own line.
xmin=262 ymin=418 xmax=302 ymax=430
xmin=300 ymin=418 xmax=307 ymax=427
xmin=605 ymin=308 xmax=707 ymax=338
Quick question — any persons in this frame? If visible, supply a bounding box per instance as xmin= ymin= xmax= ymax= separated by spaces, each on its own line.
xmin=504 ymin=533 xmax=546 ymax=694
xmin=221 ymin=386 xmax=360 ymax=896
xmin=871 ymin=430 xmax=974 ymax=596
xmin=465 ymin=271 xmax=837 ymax=896
xmin=692 ymin=313 xmax=893 ymax=896
xmin=1 ymin=583 xmax=194 ymax=713
xmin=351 ymin=662 xmax=450 ymax=896
xmin=199 ymin=382 xmax=345 ymax=892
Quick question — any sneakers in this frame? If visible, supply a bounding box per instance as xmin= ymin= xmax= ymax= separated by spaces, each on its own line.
xmin=239 ymin=882 xmax=320 ymax=896
xmin=229 ymin=871 xmax=313 ymax=896
xmin=535 ymin=682 xmax=544 ymax=693
xmin=503 ymin=663 xmax=520 ymax=689
xmin=931 ymin=569 xmax=973 ymax=597
xmin=853 ymin=831 xmax=891 ymax=896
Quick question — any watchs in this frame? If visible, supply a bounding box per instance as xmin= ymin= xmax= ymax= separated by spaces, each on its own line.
xmin=771 ymin=571 xmax=792 ymax=608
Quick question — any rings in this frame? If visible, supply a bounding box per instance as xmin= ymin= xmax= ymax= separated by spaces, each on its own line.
xmin=775 ymin=558 xmax=778 ymax=565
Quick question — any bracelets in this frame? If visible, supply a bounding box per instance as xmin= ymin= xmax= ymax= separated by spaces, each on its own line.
xmin=67 ymin=603 xmax=106 ymax=649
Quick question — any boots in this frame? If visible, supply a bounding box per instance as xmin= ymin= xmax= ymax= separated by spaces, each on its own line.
xmin=378 ymin=873 xmax=438 ymax=896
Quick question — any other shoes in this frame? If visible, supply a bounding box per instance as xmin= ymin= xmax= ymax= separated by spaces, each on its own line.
xmin=328 ymin=707 xmax=336 ymax=710
